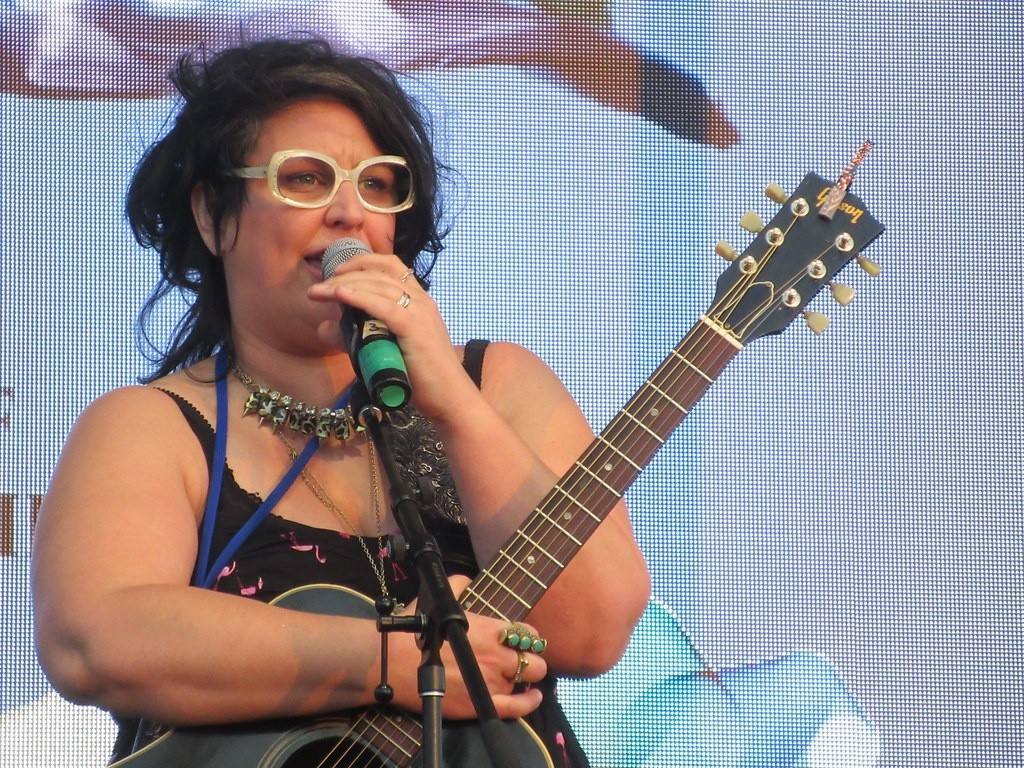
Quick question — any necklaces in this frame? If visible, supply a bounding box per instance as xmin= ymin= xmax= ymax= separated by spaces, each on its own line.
xmin=232 ymin=363 xmax=406 ymax=615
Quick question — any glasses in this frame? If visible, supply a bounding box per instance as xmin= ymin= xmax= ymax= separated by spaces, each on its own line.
xmin=226 ymin=149 xmax=416 ymax=214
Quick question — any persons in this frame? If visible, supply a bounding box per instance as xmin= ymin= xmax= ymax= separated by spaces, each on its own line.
xmin=31 ymin=31 xmax=651 ymax=768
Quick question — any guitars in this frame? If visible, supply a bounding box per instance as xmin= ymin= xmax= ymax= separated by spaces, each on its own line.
xmin=106 ymin=136 xmax=892 ymax=768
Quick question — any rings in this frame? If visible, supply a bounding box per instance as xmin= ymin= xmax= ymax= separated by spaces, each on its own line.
xmin=512 ymin=649 xmax=528 ymax=683
xmin=500 ymin=621 xmax=547 ymax=654
xmin=396 ymin=292 xmax=411 ymax=308
xmin=399 ymin=268 xmax=414 ymax=284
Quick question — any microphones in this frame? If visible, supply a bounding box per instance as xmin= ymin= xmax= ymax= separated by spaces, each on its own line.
xmin=322 ymin=237 xmax=412 ymax=412
